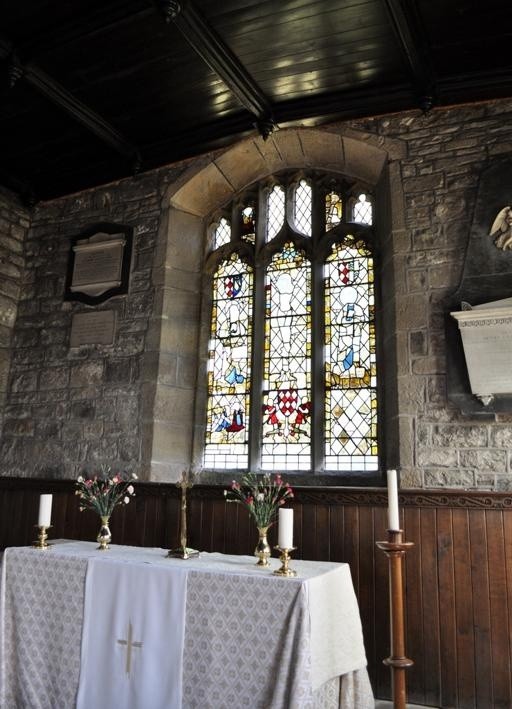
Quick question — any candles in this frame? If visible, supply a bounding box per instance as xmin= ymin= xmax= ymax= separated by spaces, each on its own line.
xmin=38 ymin=493 xmax=52 ymax=527
xmin=387 ymin=468 xmax=399 ymax=534
xmin=278 ymin=507 xmax=293 ymax=550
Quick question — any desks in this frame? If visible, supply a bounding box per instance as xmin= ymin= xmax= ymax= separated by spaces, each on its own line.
xmin=0 ymin=539 xmax=374 ymax=709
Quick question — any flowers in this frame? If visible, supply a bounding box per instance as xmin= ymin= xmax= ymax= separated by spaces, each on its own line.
xmin=75 ymin=463 xmax=138 ymax=516
xmin=222 ymin=471 xmax=293 ymax=528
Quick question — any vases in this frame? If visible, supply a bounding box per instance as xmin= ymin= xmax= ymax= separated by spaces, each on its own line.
xmin=96 ymin=516 xmax=112 ymax=548
xmin=253 ymin=523 xmax=271 ymax=566
